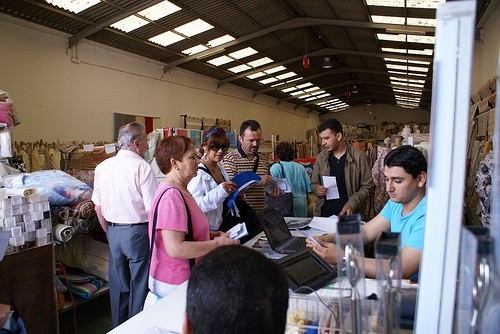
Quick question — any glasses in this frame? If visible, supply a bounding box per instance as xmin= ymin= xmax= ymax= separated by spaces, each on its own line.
xmin=207 ymin=141 xmax=229 ymax=151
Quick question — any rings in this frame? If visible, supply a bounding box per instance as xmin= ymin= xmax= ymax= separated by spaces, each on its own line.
xmin=228 ymin=189 xmax=232 ymax=192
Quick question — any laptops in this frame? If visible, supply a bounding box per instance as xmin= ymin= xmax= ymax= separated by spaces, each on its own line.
xmin=256 ymin=204 xmax=307 ymax=254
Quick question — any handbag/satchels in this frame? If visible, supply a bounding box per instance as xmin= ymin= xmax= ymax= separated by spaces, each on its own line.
xmin=140 ymin=291 xmax=161 ymax=311
xmin=268 ymin=163 xmax=293 ymax=215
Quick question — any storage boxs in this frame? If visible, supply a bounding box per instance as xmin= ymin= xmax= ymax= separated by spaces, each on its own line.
xmin=82 ymin=144 xmax=117 ymax=165
xmin=0 ymin=189 xmax=52 ymax=254
xmin=156 ymin=129 xmax=237 ymax=148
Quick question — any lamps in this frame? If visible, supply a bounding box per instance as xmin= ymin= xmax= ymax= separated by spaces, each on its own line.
xmin=344 ymin=73 xmax=350 ymax=97
xmin=322 ymin=48 xmax=332 ymax=69
xmin=196 ymin=48 xmax=226 ymax=61
xmin=303 ymin=19 xmax=310 ymax=70
xmin=352 ymin=81 xmax=360 ymax=95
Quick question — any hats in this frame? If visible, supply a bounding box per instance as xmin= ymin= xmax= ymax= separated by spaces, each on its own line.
xmin=226 ymin=171 xmax=261 ymax=217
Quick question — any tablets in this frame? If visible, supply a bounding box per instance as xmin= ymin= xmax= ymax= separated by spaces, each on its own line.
xmin=274 ymin=248 xmax=338 ymax=293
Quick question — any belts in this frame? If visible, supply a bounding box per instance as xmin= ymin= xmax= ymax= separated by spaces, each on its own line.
xmin=108 ymin=222 xmax=148 ymax=226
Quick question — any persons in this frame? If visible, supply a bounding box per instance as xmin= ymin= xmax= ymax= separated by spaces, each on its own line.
xmin=269 ymin=139 xmax=313 ymax=218
xmin=310 ymin=118 xmax=376 ymax=219
xmin=148 ymin=136 xmax=241 ymax=299
xmin=305 ymin=144 xmax=427 ymax=331
xmin=365 ymin=137 xmax=403 ymax=219
xmin=91 ymin=122 xmax=159 ymax=331
xmin=222 ymin=120 xmax=285 ymax=215
xmin=187 ymin=124 xmax=237 ymax=232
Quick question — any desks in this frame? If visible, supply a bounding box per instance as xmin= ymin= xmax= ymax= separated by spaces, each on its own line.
xmin=104 ymin=217 xmax=413 ymax=334
xmin=0 ymin=240 xmax=58 ymax=334
